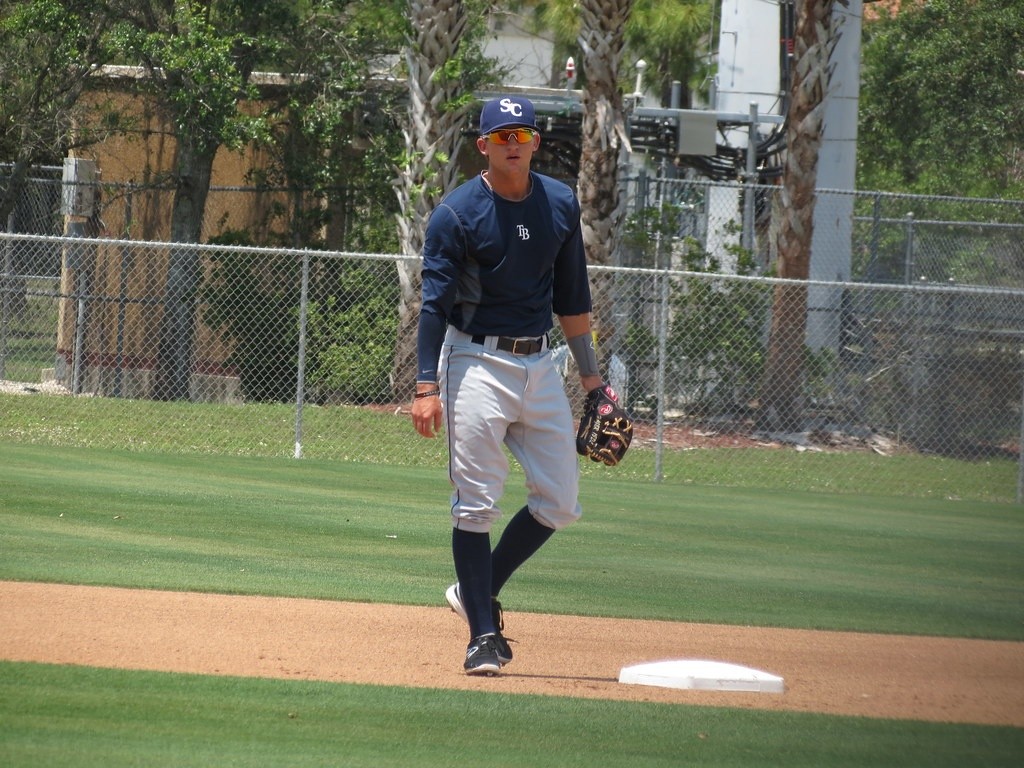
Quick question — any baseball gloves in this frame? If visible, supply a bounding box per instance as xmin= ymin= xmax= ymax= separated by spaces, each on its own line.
xmin=574 ymin=384 xmax=636 ymax=468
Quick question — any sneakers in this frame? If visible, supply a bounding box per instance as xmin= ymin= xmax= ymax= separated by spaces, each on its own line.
xmin=445 ymin=581 xmax=519 ymax=666
xmin=463 ymin=632 xmax=500 ymax=676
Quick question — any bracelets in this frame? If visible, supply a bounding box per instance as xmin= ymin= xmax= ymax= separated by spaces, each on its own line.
xmin=415 ymin=389 xmax=440 ymax=398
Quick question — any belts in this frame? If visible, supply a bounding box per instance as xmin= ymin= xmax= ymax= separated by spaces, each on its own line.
xmin=471 ymin=332 xmax=550 ymax=356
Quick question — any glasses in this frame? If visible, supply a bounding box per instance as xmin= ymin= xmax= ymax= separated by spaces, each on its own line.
xmin=483 ymin=127 xmax=537 ymax=144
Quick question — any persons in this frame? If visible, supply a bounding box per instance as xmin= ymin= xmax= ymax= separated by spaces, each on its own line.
xmin=411 ymin=95 xmax=633 ymax=676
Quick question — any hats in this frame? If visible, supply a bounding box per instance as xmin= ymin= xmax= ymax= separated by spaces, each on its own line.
xmin=479 ymin=96 xmax=541 ymax=135
